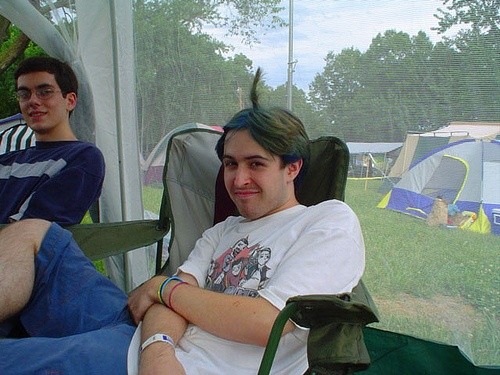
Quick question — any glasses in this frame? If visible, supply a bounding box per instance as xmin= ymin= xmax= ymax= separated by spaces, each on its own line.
xmin=14 ymin=88 xmax=63 ymax=102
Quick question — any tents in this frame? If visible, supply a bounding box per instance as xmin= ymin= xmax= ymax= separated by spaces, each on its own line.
xmin=143 ymin=122 xmax=215 ymax=188
xmin=346 ymin=122 xmax=500 ymax=236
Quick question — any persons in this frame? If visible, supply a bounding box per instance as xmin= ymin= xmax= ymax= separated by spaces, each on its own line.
xmin=0 ymin=56 xmax=106 ymax=227
xmin=0 ymin=68 xmax=365 ymax=375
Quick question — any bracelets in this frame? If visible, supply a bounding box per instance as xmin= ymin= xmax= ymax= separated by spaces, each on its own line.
xmin=158 ymin=277 xmax=188 ymax=310
xmin=139 ymin=334 xmax=176 ymax=352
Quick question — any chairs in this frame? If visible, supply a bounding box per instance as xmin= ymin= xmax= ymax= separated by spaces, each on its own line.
xmin=63 ymin=127 xmax=380 ymax=375
